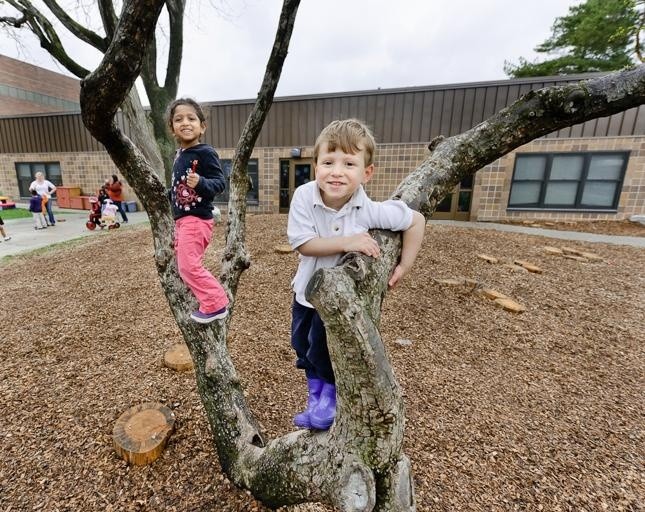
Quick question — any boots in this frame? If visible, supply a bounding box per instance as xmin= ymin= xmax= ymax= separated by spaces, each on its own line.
xmin=293 ymin=379 xmax=323 ymax=427
xmin=309 ymin=382 xmax=337 ymax=430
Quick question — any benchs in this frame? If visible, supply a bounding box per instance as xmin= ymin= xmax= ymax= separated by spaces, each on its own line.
xmin=0 ymin=203 xmax=16 ymax=211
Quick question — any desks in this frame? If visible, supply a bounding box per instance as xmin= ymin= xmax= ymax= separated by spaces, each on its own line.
xmin=0 ymin=195 xmax=9 ymax=204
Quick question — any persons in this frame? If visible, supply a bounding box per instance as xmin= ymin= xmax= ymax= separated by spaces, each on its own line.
xmin=27 ymin=171 xmax=57 ymax=226
xmin=285 ymin=117 xmax=427 ymax=430
xmin=28 ymin=189 xmax=48 ymax=231
xmin=0 ymin=215 xmax=11 ymax=244
xmin=167 ymin=96 xmax=232 ymax=325
xmin=85 ymin=173 xmax=128 ymax=231
xmin=39 ymin=192 xmax=50 ymax=227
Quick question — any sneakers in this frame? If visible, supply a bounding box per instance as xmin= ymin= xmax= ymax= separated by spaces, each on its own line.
xmin=5 ymin=237 xmax=11 ymax=241
xmin=189 ymin=306 xmax=228 ymax=324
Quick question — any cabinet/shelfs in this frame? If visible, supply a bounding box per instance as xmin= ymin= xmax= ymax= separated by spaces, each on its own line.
xmin=56 ymin=187 xmax=83 ymax=209
xmin=80 ymin=196 xmax=98 ymax=210
